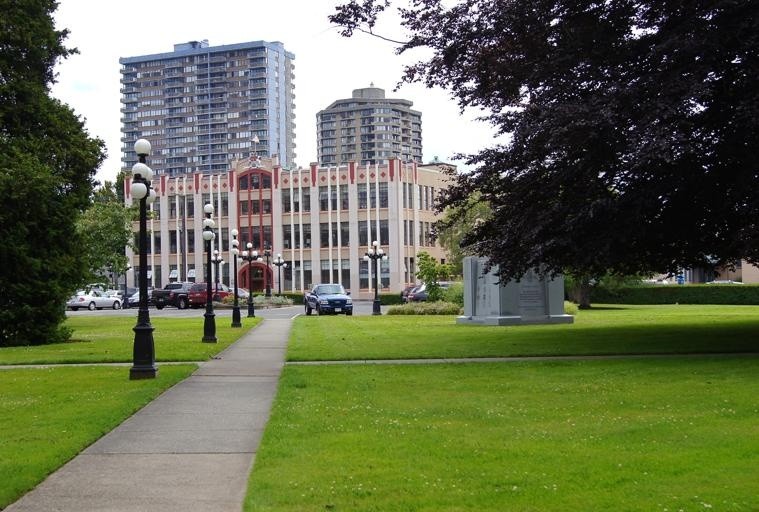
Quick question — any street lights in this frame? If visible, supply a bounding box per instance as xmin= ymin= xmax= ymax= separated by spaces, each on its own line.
xmin=231 ymin=229 xmax=242 ymax=327
xmin=272 ymin=254 xmax=287 ymax=294
xmin=239 ymin=242 xmax=258 ymax=318
xmin=129 ymin=137 xmax=160 ymax=380
xmin=124 ymin=263 xmax=133 ymax=308
xmin=211 ymin=249 xmax=225 ymax=301
xmin=363 ymin=240 xmax=388 ymax=315
xmin=201 ymin=200 xmax=218 ymax=343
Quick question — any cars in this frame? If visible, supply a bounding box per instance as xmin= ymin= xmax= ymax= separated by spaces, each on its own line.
xmin=128 ymin=281 xmax=250 ymax=310
xmin=305 ymin=284 xmax=353 ymax=316
xmin=408 ymin=281 xmax=449 ymax=302
xmin=402 ymin=280 xmax=429 ymax=302
xmin=66 ymin=283 xmax=125 ymax=310
